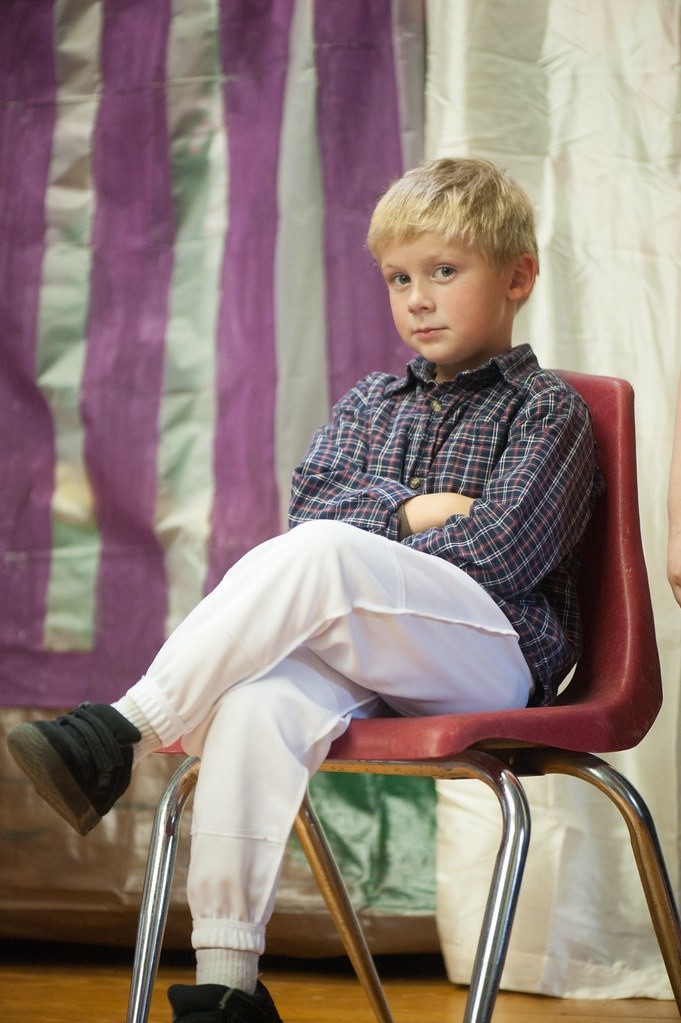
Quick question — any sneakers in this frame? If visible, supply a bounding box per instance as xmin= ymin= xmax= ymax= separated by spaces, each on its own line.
xmin=167 ymin=976 xmax=285 ymax=1023
xmin=8 ymin=701 xmax=142 ymax=837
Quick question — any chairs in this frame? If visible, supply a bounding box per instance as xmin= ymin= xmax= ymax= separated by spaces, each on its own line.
xmin=126 ymin=367 xmax=679 ymax=1022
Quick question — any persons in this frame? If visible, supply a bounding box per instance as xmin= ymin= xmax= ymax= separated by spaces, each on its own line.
xmin=0 ymin=153 xmax=610 ymax=1023
